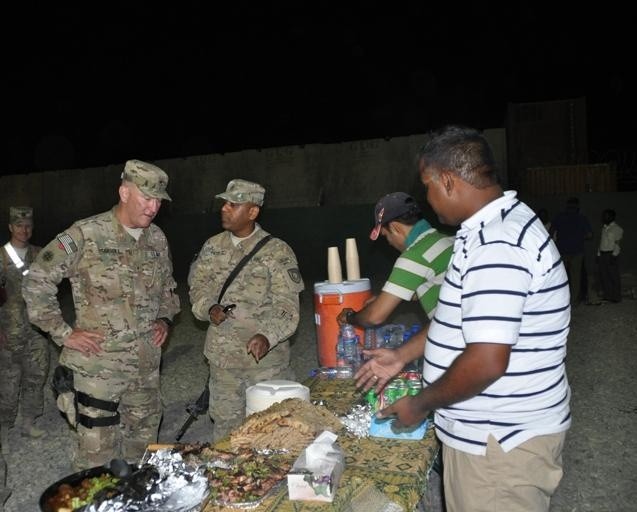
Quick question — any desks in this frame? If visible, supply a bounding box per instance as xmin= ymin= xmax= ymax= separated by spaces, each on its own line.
xmin=182 ymin=367 xmax=446 ymax=512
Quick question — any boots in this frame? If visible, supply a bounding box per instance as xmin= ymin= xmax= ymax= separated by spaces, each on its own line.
xmin=20 ymin=418 xmax=47 ymax=440
xmin=0 ymin=427 xmax=11 ymax=456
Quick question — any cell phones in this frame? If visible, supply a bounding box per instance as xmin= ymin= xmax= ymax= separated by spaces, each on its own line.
xmin=222 ymin=304 xmax=236 ymax=313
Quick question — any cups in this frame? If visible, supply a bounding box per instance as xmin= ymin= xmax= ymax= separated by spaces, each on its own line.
xmin=345 ymin=237 xmax=361 ymax=281
xmin=327 ymin=245 xmax=344 ymax=286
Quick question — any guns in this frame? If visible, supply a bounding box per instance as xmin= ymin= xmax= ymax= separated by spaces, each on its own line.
xmin=175 ymin=376 xmax=209 ymax=441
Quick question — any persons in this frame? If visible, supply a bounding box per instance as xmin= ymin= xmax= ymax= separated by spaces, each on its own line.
xmin=0 ymin=206 xmax=47 ymax=455
xmin=188 ymin=180 xmax=305 ymax=443
xmin=337 ymin=191 xmax=456 ymax=327
xmin=536 ymin=206 xmax=558 ymax=246
xmin=22 ymin=159 xmax=180 ymax=476
xmin=598 ymin=209 xmax=624 ymax=304
xmin=559 ymin=195 xmax=599 ymax=305
xmin=351 ymin=129 xmax=573 ymax=512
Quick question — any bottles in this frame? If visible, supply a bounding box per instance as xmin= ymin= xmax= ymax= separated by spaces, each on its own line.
xmin=353 ymin=335 xmax=363 ymax=366
xmin=310 ymin=366 xmax=353 ymax=379
xmin=365 ymin=323 xmax=421 ymax=371
xmin=336 ymin=336 xmax=345 ymax=367
xmin=342 ymin=320 xmax=356 ymax=365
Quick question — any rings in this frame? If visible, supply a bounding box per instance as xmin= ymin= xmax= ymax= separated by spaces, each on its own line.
xmin=372 ymin=374 xmax=378 ymax=379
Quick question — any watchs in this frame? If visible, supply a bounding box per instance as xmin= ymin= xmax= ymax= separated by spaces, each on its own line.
xmin=346 ymin=310 xmax=356 ymax=324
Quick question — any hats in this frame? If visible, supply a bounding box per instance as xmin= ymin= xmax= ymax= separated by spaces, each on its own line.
xmin=120 ymin=159 xmax=172 ymax=202
xmin=369 ymin=191 xmax=418 ymax=241
xmin=9 ymin=206 xmax=34 ymax=227
xmin=214 ymin=178 xmax=266 ymax=207
